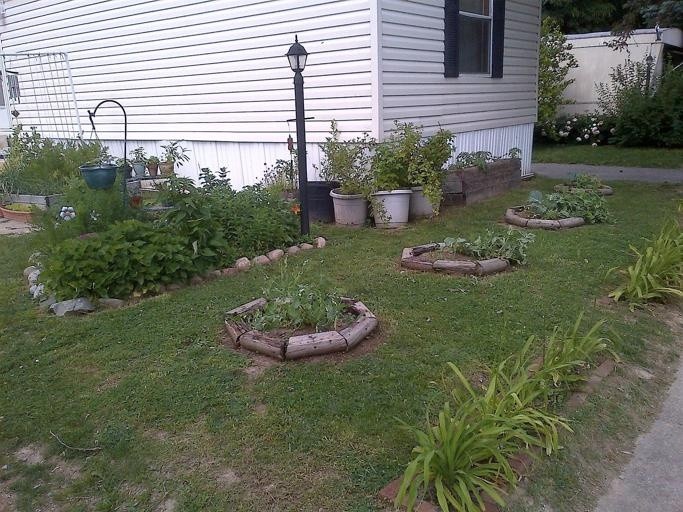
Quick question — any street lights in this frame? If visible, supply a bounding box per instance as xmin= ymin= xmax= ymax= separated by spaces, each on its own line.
xmin=284 ymin=34 xmax=310 ymax=239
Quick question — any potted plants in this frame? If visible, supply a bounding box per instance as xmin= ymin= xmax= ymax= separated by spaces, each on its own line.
xmin=79 ymin=146 xmax=118 ymax=189
xmin=409 ymin=122 xmax=456 ymax=217
xmin=115 ymin=157 xmax=132 ymax=178
xmin=0 ymin=194 xmax=35 ymax=222
xmin=145 ymin=156 xmax=159 ymax=177
xmin=306 ymin=126 xmax=369 ymax=229
xmin=142 ymin=182 xmax=173 ymax=212
xmin=372 ymin=121 xmax=417 ymax=229
xmin=158 ymin=139 xmax=191 ymax=175
xmin=128 ymin=146 xmax=147 ymax=175
xmin=269 ymin=148 xmax=299 ymax=199
